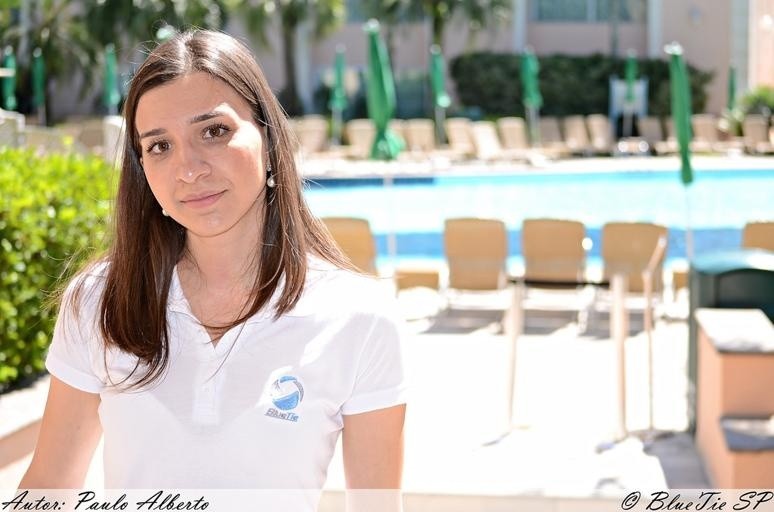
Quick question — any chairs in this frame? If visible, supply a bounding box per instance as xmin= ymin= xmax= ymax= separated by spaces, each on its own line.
xmin=292 ymin=105 xmax=774 ymax=320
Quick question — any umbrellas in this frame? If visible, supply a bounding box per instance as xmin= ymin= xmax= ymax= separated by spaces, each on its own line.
xmin=726 ymin=61 xmax=737 ymax=112
xmin=362 ymin=18 xmax=406 ymax=231
xmin=327 ymin=40 xmax=349 ymax=144
xmin=520 ymin=42 xmax=542 ymax=139
xmin=31 ymin=43 xmax=46 ymax=121
xmin=3 ymin=44 xmax=17 ymax=109
xmin=428 ymin=44 xmax=451 ymax=148
xmin=665 ymin=45 xmax=695 ymax=187
xmin=101 ymin=42 xmax=122 ymax=112
xmin=623 ymin=48 xmax=637 ymax=104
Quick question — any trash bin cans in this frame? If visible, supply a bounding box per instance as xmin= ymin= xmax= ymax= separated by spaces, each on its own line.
xmin=688 ymin=249 xmax=774 ymax=434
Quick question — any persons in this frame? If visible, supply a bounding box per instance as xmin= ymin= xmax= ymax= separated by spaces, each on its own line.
xmin=12 ymin=28 xmax=413 ymax=512
